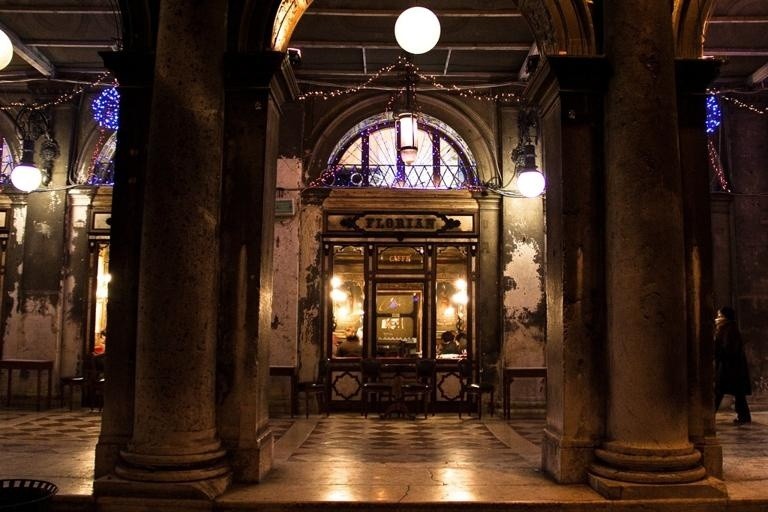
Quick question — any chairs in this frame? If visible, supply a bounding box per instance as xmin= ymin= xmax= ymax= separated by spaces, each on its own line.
xmin=295 ymin=357 xmax=331 ymax=420
xmin=89 ymin=353 xmax=105 ymax=413
xmin=359 ymin=356 xmax=494 ymax=422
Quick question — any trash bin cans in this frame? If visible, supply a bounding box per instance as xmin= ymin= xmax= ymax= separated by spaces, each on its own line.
xmin=0 ymin=478 xmax=59 ymax=512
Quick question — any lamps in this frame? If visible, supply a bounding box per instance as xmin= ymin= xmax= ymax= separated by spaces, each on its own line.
xmin=515 ymin=144 xmax=545 ymax=198
xmin=397 ymin=63 xmax=418 ymax=167
xmin=0 ymin=27 xmax=12 ymax=71
xmin=393 ymin=6 xmax=442 ymax=55
xmin=10 ymin=138 xmax=43 ymax=192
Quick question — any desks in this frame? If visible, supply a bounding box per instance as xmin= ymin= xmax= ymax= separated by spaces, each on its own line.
xmin=0 ymin=357 xmax=54 ymax=412
xmin=269 ymin=364 xmax=297 ymax=418
xmin=501 ymin=366 xmax=546 ymax=420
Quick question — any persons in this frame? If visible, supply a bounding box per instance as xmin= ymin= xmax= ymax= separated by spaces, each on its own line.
xmin=713 ymin=305 xmax=755 ymax=424
xmin=330 ymin=312 xmax=467 ymax=357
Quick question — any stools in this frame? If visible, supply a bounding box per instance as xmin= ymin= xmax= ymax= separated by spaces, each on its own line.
xmin=58 ymin=374 xmax=86 ymax=413
